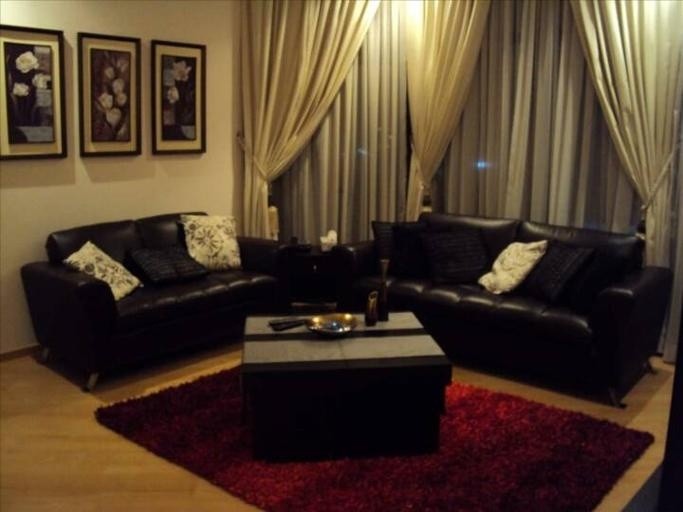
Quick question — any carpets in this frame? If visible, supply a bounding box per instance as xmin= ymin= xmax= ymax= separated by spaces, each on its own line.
xmin=93 ymin=367 xmax=655 ymax=511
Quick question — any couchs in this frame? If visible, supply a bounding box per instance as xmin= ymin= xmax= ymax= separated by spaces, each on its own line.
xmin=354 ymin=212 xmax=674 ymax=405
xmin=20 ymin=209 xmax=298 ymax=390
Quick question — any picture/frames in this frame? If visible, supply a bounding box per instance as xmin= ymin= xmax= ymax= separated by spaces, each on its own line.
xmin=150 ymin=39 xmax=206 ymax=154
xmin=77 ymin=31 xmax=141 ymax=157
xmin=0 ymin=24 xmax=67 ymax=160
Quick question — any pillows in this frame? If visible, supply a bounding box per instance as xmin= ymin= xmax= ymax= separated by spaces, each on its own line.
xmin=371 ymin=219 xmax=430 ymax=273
xmin=65 ymin=237 xmax=143 ymax=299
xmin=478 ymin=236 xmax=548 ymax=295
xmin=130 ymin=245 xmax=209 ymax=285
xmin=179 ymin=214 xmax=240 ymax=269
xmin=416 ymin=222 xmax=487 ymax=289
xmin=520 ymin=245 xmax=596 ymax=300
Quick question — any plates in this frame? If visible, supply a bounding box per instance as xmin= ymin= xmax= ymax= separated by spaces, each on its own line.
xmin=309 ymin=313 xmax=358 ymax=334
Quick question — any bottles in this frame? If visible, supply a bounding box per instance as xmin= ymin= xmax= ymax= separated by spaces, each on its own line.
xmin=328 ymin=230 xmax=338 ymax=247
xmin=366 ymin=260 xmax=390 ymax=326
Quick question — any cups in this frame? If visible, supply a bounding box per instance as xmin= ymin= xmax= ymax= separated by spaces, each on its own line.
xmin=321 ymin=237 xmax=334 ymax=252
